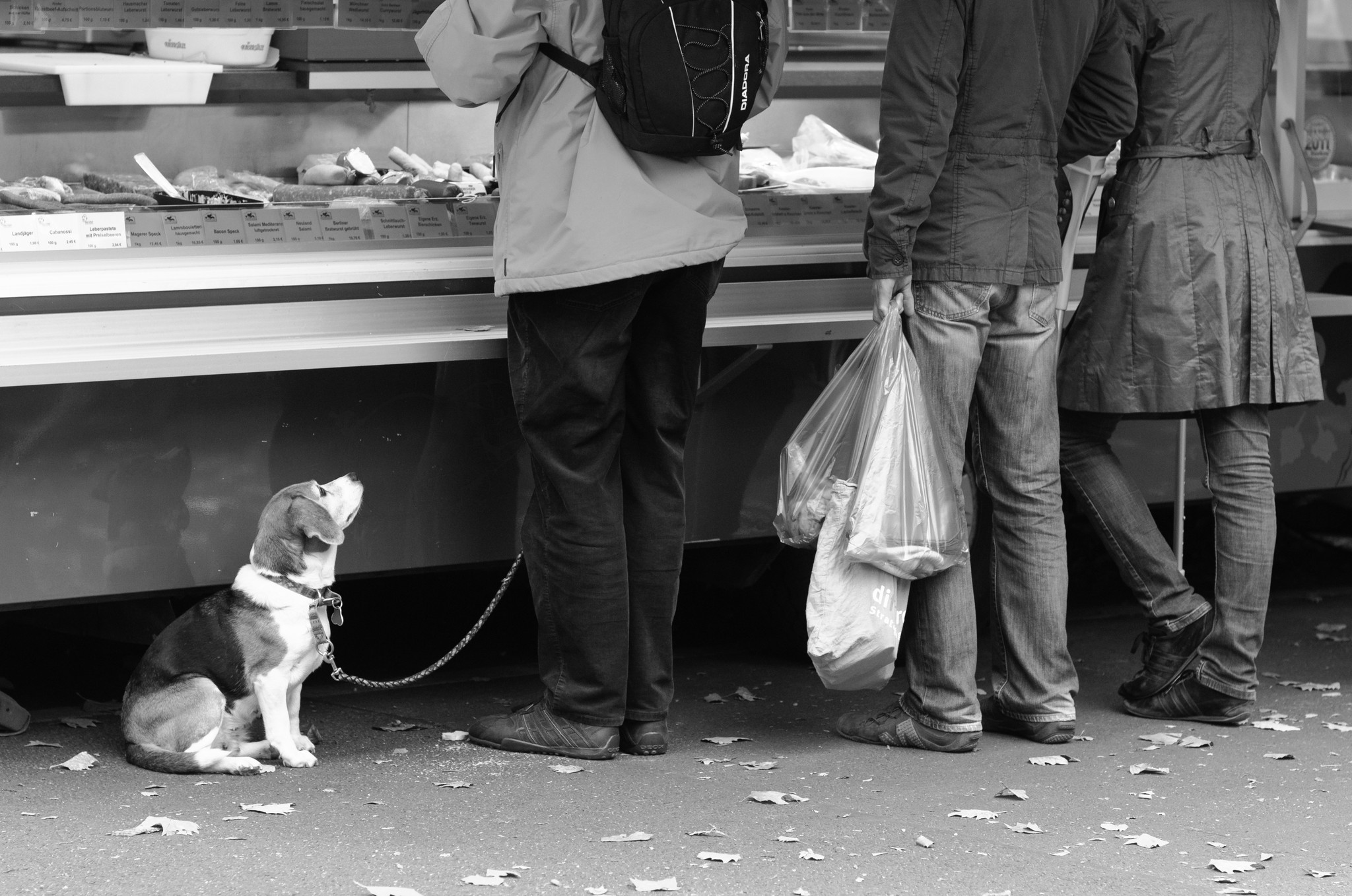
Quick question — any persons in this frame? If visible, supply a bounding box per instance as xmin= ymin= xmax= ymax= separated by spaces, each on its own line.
xmin=411 ymin=1 xmax=793 ymax=761
xmin=1054 ymin=0 xmax=1329 ymax=730
xmin=835 ymin=0 xmax=1139 ymax=752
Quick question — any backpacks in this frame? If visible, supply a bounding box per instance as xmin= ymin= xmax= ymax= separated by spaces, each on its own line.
xmin=588 ymin=1 xmax=769 ymax=164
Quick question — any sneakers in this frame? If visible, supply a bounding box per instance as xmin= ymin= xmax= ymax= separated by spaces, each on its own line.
xmin=835 ymin=700 xmax=982 ymax=753
xmin=464 ymin=693 xmax=621 ymax=762
xmin=1116 ymin=607 xmax=1213 ymax=701
xmin=1121 ymin=672 xmax=1256 ymax=728
xmin=621 ymin=716 xmax=669 ymax=756
xmin=977 ymin=693 xmax=1077 ymax=743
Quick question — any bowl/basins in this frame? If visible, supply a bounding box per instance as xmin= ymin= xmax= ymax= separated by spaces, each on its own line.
xmin=152 ymin=186 xmax=266 ymax=213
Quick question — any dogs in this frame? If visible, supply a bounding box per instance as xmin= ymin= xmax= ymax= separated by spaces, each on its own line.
xmin=121 ymin=471 xmax=364 ymax=776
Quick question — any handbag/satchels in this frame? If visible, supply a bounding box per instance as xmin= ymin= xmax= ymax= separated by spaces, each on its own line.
xmin=766 ymin=298 xmax=966 ymax=696
